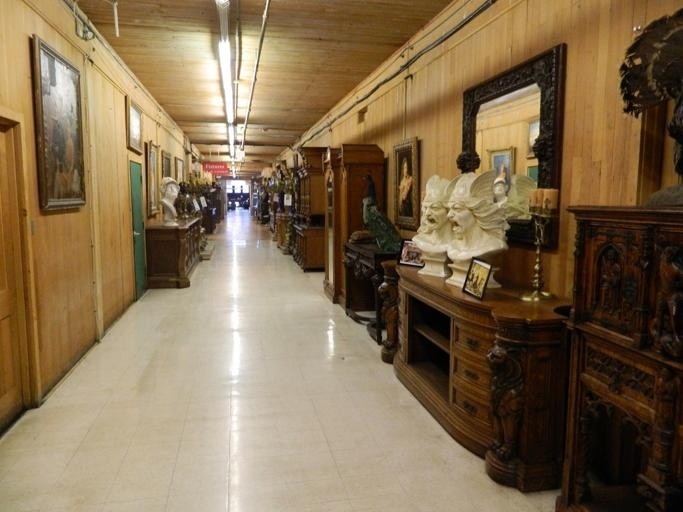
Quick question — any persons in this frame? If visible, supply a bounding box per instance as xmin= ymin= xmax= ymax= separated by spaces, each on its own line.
xmin=398 ymin=157 xmax=508 ymax=260
xmin=155 ymin=177 xmax=179 ymax=218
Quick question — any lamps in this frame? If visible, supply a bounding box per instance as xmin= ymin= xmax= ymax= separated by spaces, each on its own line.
xmin=210 ymin=0 xmax=238 ymax=158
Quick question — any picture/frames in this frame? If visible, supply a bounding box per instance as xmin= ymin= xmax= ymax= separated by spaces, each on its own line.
xmin=393 ymin=137 xmax=420 ymax=232
xmin=397 ymin=239 xmax=425 ymax=268
xmin=486 ymin=146 xmax=515 ymax=178
xmin=161 ymin=150 xmax=171 ymax=179
xmin=174 ymin=156 xmax=184 ymax=184
xmin=523 ymin=163 xmax=540 ymax=184
xmin=29 ymin=33 xmax=86 ymax=214
xmin=146 ymin=141 xmax=160 ymax=219
xmin=525 ymin=114 xmax=540 ymax=159
xmin=125 ymin=95 xmax=143 ymax=156
xmin=462 ymin=257 xmax=491 ymax=300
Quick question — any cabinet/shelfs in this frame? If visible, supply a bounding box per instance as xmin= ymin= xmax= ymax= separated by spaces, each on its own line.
xmin=556 ymin=204 xmax=681 ymax=512
xmin=295 ymin=146 xmax=327 ymax=217
xmin=144 ymin=218 xmax=202 ymax=288
xmin=322 ymin=144 xmax=384 ymax=312
xmin=274 ymin=213 xmax=292 ymax=254
xmin=393 ymin=264 xmax=574 ymax=496
xmin=292 ymin=224 xmax=326 ymax=272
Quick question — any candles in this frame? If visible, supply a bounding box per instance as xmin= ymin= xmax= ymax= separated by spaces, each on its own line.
xmin=542 ymin=188 xmax=559 ymax=210
xmin=527 ymin=186 xmax=541 ymax=211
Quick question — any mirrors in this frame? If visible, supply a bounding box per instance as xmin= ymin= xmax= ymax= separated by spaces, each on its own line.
xmin=462 ymin=42 xmax=565 ymax=251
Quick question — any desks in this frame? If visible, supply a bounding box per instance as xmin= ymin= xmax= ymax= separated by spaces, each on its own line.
xmin=342 ymin=241 xmax=401 ymax=344
